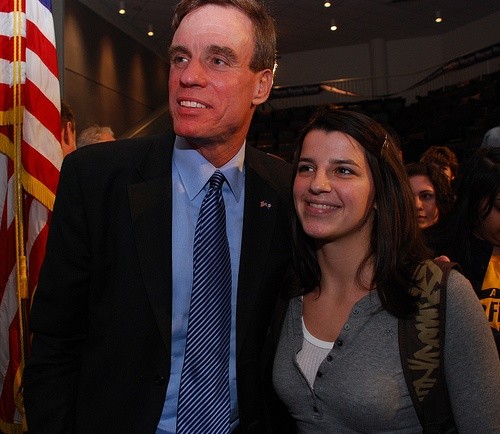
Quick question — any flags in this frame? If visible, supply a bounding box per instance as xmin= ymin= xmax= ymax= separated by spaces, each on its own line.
xmin=0 ymin=0 xmax=65 ymax=424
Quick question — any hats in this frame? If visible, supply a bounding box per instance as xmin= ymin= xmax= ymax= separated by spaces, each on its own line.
xmin=481 ymin=127 xmax=500 ymax=148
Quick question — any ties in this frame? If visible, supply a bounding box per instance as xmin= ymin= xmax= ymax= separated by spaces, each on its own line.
xmin=176 ymin=170 xmax=233 ymax=434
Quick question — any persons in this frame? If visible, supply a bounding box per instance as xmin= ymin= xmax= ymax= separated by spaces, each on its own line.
xmin=403 ymin=125 xmax=500 ymax=360
xmin=19 ymin=0 xmax=306 ymax=434
xmin=270 ymin=108 xmax=500 ymax=434
xmin=60 ymin=101 xmax=77 ymax=158
xmin=76 ymin=125 xmax=116 ymax=149
